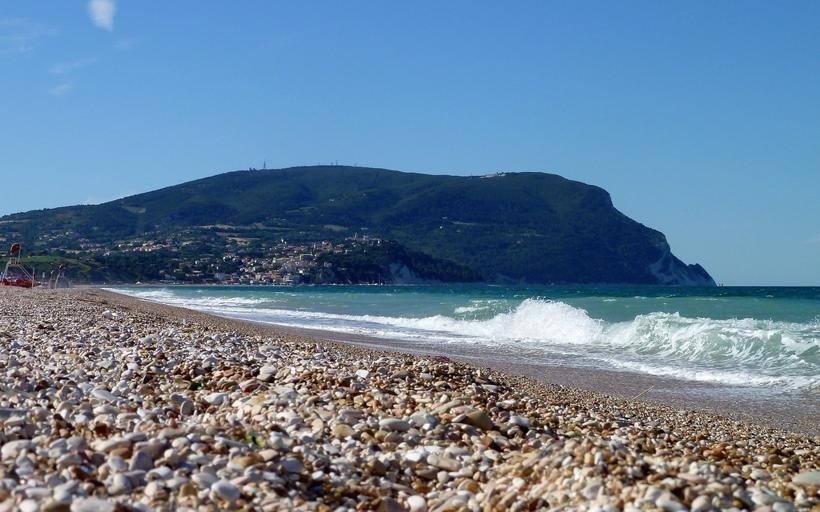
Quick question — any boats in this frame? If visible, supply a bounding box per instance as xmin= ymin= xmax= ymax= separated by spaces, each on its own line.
xmin=0 ymin=272 xmax=41 ymax=288
